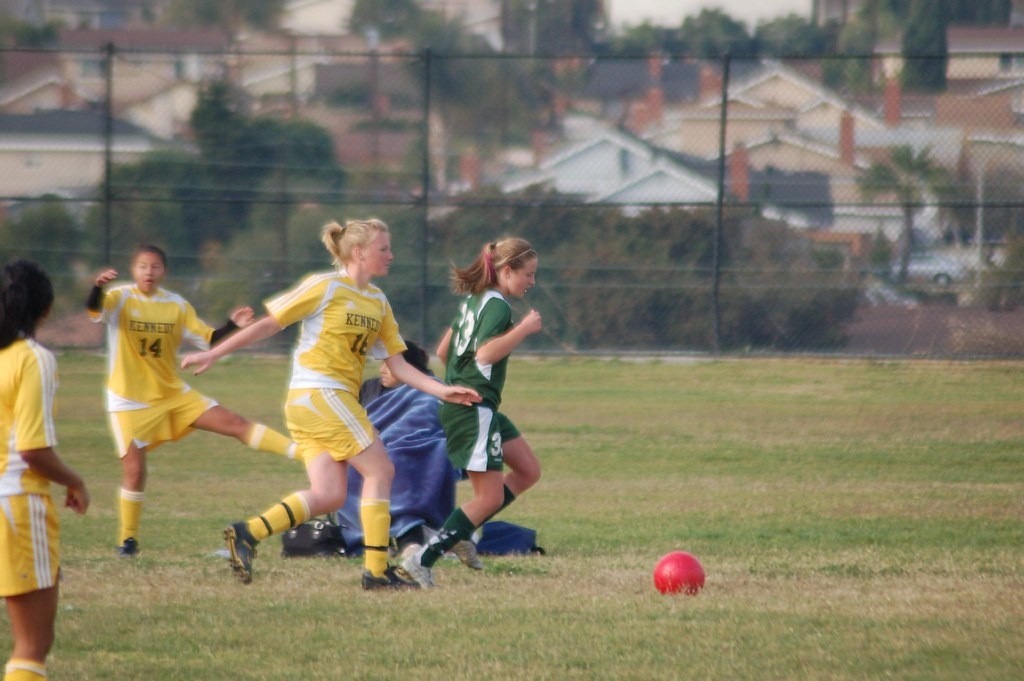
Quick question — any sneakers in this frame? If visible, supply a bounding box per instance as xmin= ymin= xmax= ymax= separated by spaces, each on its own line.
xmin=118 ymin=537 xmax=140 ymax=557
xmin=362 ymin=561 xmax=421 ymax=592
xmin=452 ymin=539 xmax=484 ymax=571
xmin=397 ymin=556 xmax=434 ymax=591
xmin=224 ymin=520 xmax=259 ymax=585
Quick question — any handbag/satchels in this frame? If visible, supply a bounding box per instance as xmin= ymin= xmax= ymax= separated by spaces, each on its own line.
xmin=281 ymin=510 xmax=350 ymax=558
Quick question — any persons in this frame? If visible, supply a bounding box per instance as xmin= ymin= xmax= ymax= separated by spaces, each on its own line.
xmin=336 ymin=339 xmax=478 ymax=568
xmin=179 ymin=218 xmax=482 ymax=590
xmin=85 ymin=243 xmax=303 ymax=556
xmin=0 ymin=258 xmax=90 ymax=681
xmin=398 ymin=238 xmax=543 ymax=591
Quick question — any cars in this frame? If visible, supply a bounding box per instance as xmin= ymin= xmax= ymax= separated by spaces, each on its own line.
xmin=889 ymin=248 xmax=967 ymax=286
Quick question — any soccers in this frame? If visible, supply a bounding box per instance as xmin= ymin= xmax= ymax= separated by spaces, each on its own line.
xmin=654 ymin=552 xmax=707 ymax=596
